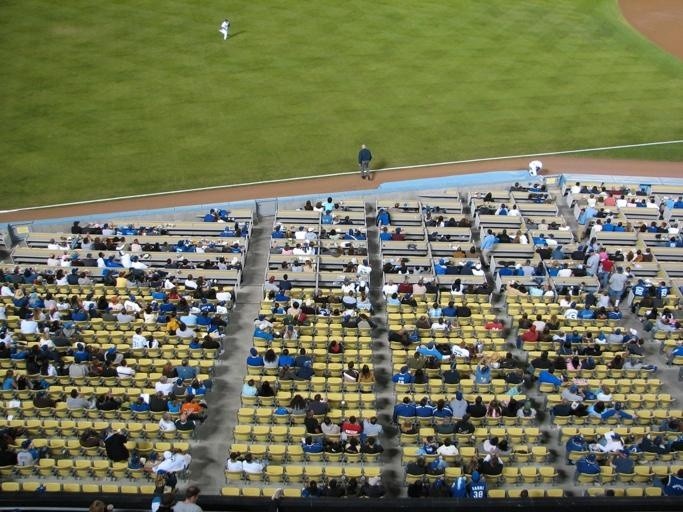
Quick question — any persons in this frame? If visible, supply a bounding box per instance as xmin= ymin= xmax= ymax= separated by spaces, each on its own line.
xmin=219 ymin=19 xmax=230 ymax=40
xmin=357 ymin=144 xmax=373 ymax=181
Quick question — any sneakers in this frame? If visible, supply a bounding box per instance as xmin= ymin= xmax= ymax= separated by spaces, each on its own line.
xmin=361 ymin=174 xmax=371 ymax=182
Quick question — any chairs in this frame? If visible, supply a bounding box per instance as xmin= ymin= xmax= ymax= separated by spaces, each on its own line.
xmin=265 ymin=182 xmax=683 ymax=294
xmin=0 ymin=208 xmax=255 ymax=294
xmin=0 ymin=294 xmax=683 ymax=512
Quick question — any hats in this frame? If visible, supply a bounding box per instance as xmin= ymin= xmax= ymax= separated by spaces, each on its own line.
xmin=15 ymin=211 xmax=240 ymax=467
xmin=561 ymin=249 xmax=646 ymax=411
xmin=611 ymin=433 xmax=621 ymax=440
xmin=368 ymin=477 xmax=383 ymax=486
xmin=454 ymin=477 xmax=465 ymax=489
xmin=250 ymin=209 xmax=369 ymax=368
xmin=586 ymin=453 xmax=597 ymax=463
xmin=471 ymin=470 xmax=479 ymax=483
xmin=379 ymin=205 xmax=532 ymax=468
xmin=575 ymin=435 xmax=585 ymax=445
xmin=619 ymin=449 xmax=630 ymax=458
